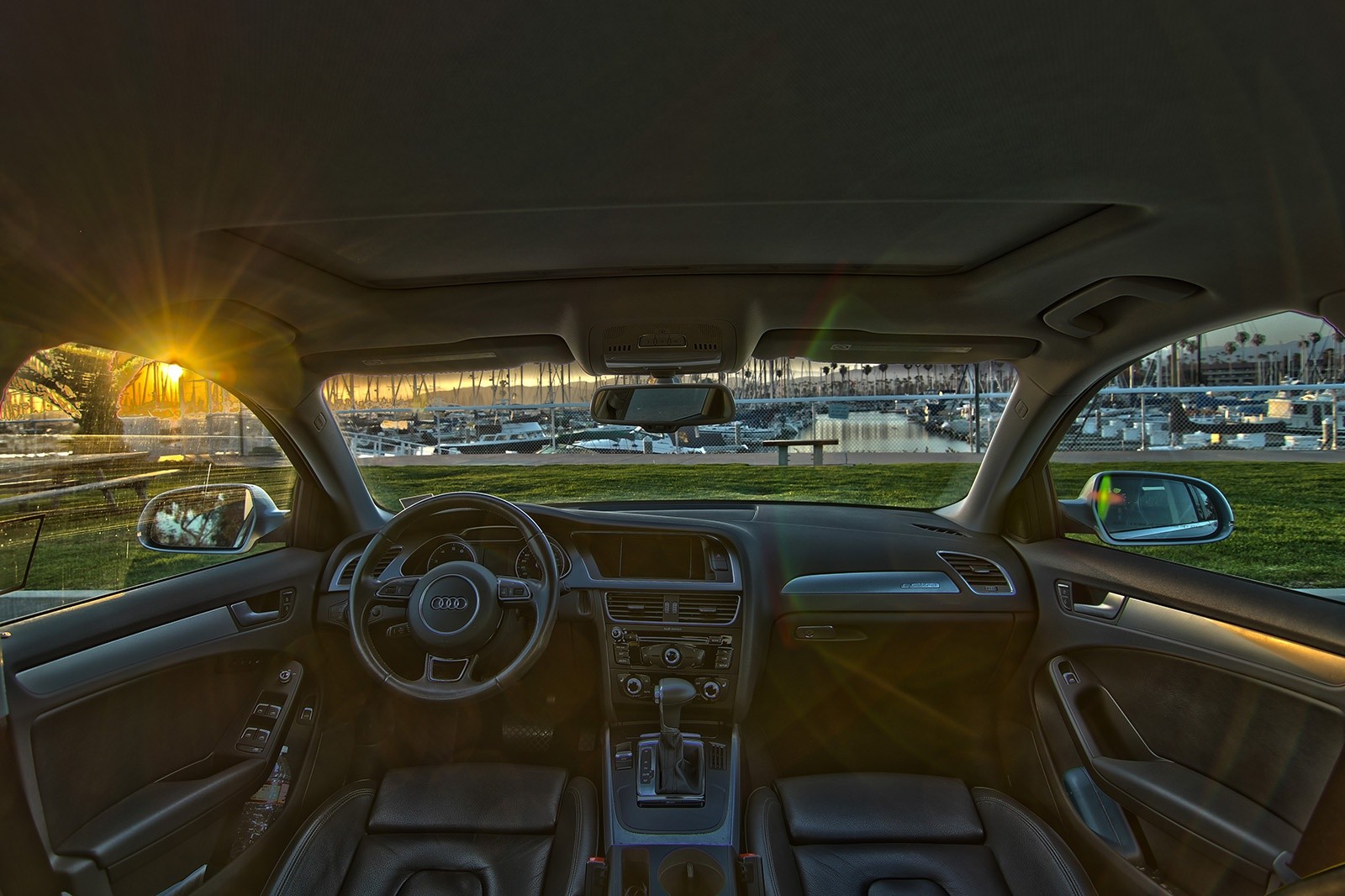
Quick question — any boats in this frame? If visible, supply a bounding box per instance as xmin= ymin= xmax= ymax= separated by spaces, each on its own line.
xmin=0 ymin=358 xmax=1344 ymax=476
